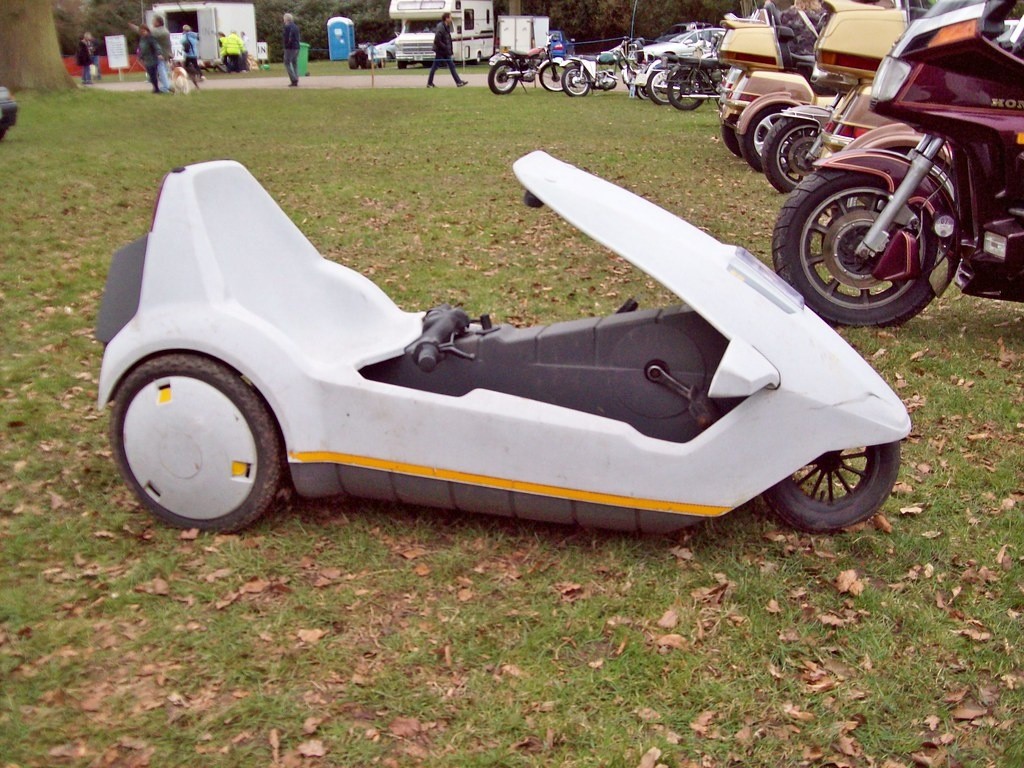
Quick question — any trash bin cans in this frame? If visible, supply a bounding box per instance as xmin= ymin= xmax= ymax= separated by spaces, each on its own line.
xmin=297 ymin=42 xmax=310 ymax=76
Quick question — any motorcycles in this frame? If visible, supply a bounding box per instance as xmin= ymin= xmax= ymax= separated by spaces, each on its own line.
xmin=348 ymin=42 xmax=386 ymax=69
xmin=488 ymin=33 xmax=574 ymax=95
xmin=559 ymin=36 xmax=642 ymax=97
xmin=630 ymin=0 xmax=1024 ymax=328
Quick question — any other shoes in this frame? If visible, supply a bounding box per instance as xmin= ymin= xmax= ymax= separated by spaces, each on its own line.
xmin=456 ymin=80 xmax=468 ymax=87
xmin=288 ymin=80 xmax=298 ymax=87
xmin=426 ymin=84 xmax=436 ymax=88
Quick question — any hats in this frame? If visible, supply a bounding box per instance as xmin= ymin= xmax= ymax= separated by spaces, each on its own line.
xmin=182 ymin=24 xmax=191 ymax=31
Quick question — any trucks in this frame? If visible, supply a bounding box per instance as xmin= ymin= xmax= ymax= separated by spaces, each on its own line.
xmin=497 ymin=15 xmax=550 ymax=56
xmin=389 ymin=0 xmax=495 ymax=70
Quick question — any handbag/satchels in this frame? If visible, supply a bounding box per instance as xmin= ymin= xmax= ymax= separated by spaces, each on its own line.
xmin=182 ymin=39 xmax=193 ymax=53
xmin=89 ymin=64 xmax=97 ymax=76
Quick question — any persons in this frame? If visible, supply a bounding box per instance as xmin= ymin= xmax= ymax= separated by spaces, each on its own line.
xmin=781 ymin=0 xmax=827 ymax=55
xmin=83 ymin=32 xmax=101 ymax=81
xmin=75 ymin=36 xmax=93 ymax=85
xmin=283 ymin=14 xmax=300 ymax=87
xmin=129 ymin=16 xmax=173 ymax=92
xmin=180 ymin=25 xmax=206 ymax=81
xmin=219 ymin=31 xmax=248 ymax=73
xmin=427 ymin=13 xmax=467 ymax=88
xmin=138 ymin=24 xmax=163 ymax=94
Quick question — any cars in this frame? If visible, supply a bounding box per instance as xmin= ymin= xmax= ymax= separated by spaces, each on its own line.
xmin=642 ymin=27 xmax=726 ymax=63
xmin=550 ymin=31 xmax=575 ymax=61
xmin=655 ymin=22 xmax=712 ymax=43
xmin=375 ymin=36 xmax=397 ymax=62
xmin=0 ymin=86 xmax=18 ymax=141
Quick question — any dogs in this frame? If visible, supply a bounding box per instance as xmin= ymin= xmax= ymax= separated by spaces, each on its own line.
xmin=172 ymin=67 xmax=190 ymax=96
